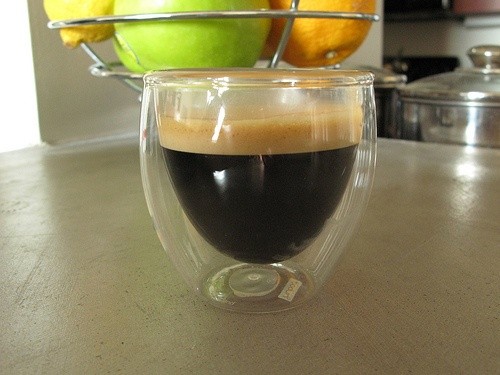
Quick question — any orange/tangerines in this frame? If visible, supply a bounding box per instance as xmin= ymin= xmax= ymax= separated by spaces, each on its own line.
xmin=269 ymin=0 xmax=376 ymax=67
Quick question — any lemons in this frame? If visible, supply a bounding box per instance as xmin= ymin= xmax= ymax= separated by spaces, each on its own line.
xmin=43 ymin=0 xmax=115 ymax=47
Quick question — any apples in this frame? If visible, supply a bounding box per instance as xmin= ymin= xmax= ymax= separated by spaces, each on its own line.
xmin=113 ymin=0 xmax=274 ymax=75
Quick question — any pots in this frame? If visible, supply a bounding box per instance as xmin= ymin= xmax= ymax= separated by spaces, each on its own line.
xmin=350 ymin=64 xmax=407 ymax=141
xmin=398 ymin=44 xmax=500 ymax=149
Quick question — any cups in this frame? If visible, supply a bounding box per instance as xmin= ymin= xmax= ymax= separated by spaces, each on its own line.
xmin=139 ymin=69 xmax=377 ymax=317
